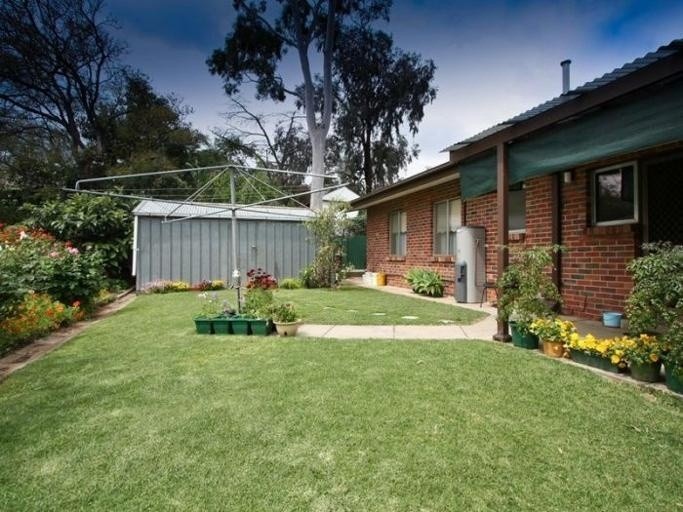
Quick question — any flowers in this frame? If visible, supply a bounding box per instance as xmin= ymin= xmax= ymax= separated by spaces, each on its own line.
xmin=528 ymin=316 xmax=668 ymax=369
xmin=193 ymin=290 xmax=245 ymax=320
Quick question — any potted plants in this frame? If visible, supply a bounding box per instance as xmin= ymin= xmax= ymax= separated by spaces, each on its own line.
xmin=245 ymin=286 xmax=302 ymax=337
xmin=481 ymin=240 xmax=569 ymax=349
xmin=626 ymin=240 xmax=683 ymax=396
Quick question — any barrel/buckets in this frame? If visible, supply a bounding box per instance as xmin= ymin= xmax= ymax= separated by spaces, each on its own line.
xmin=603 ymin=312 xmax=622 ymax=328
xmin=361 ymin=272 xmax=386 ymax=286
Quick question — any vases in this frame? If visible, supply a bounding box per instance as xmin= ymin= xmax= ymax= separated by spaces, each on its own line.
xmin=602 ymin=357 xmax=626 ymax=373
xmin=194 ymin=319 xmax=249 ymax=335
